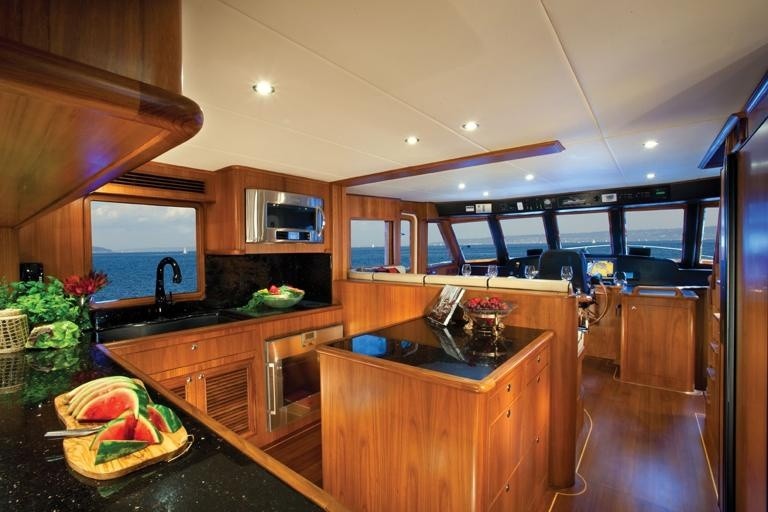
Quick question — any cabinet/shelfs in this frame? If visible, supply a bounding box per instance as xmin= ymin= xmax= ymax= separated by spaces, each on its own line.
xmin=617 ymin=287 xmax=698 ymax=396
xmin=314 ymin=314 xmax=556 ymax=511
xmin=105 ymin=315 xmax=265 ymax=455
xmin=2 ymin=1 xmax=202 ymax=230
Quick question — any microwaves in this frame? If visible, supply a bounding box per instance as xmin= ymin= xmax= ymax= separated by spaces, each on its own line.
xmin=246 ymin=188 xmax=327 ymax=244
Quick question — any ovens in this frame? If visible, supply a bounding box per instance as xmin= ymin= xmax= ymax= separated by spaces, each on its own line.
xmin=266 ymin=322 xmax=344 ymax=430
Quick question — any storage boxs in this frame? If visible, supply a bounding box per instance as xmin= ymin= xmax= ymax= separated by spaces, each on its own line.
xmin=0 ymin=309 xmax=29 ymax=355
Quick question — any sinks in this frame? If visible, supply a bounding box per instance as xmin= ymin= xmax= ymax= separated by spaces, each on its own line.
xmin=100 ymin=313 xmax=256 ymax=338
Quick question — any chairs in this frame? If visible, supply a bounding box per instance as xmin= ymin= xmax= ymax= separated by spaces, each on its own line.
xmin=533 ymin=248 xmax=595 ymax=309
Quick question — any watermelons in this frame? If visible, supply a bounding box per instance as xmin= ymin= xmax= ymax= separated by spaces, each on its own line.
xmin=64 ymin=376 xmax=182 ymax=464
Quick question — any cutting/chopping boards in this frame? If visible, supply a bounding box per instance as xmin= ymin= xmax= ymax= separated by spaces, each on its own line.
xmin=54 ymin=377 xmax=189 ymax=480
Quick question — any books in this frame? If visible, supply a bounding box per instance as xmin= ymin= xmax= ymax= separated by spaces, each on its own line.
xmin=426 ymin=323 xmax=466 ymax=362
xmin=427 ymin=284 xmax=466 ymax=326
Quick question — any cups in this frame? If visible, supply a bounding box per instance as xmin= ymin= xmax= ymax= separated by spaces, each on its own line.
xmin=561 ymin=266 xmax=573 ymax=280
xmin=461 ymin=264 xmax=472 ymax=276
xmin=488 ymin=265 xmax=498 ymax=277
xmin=525 ymin=265 xmax=536 ymax=279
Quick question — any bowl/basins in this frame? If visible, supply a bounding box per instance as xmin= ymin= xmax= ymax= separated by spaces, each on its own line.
xmin=252 ymin=288 xmax=305 ymax=309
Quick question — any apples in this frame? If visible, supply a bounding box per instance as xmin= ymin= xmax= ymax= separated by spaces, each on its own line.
xmin=468 ymin=297 xmax=509 ymax=310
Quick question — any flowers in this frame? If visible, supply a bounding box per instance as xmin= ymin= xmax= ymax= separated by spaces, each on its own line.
xmin=463 ymin=296 xmax=514 ymax=314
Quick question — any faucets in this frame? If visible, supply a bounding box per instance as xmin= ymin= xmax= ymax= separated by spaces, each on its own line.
xmin=155 ymin=258 xmax=182 ymax=307
